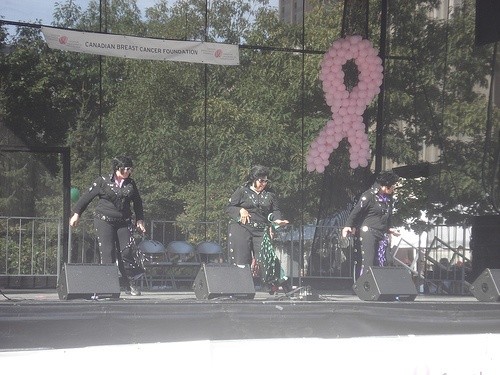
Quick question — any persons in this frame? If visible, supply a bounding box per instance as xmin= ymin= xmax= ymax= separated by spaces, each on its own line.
xmin=342 ymin=169 xmax=401 ymax=277
xmin=227 ymin=165 xmax=289 ymax=294
xmin=70 ymin=156 xmax=146 ymax=295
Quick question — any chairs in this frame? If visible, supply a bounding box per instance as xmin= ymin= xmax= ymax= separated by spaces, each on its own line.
xmin=136 ymin=239 xmax=228 ymax=292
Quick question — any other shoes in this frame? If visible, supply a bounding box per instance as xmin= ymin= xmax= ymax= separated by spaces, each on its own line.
xmin=125 ymin=285 xmax=141 ymax=295
xmin=269 ymin=279 xmax=279 ymax=295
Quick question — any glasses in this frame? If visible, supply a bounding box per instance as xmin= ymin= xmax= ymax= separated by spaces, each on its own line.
xmin=125 ymin=166 xmax=133 ymax=172
xmin=258 ymin=179 xmax=268 ymax=183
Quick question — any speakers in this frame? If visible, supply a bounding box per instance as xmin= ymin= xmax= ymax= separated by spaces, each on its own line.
xmin=352 ymin=266 xmax=418 ymax=301
xmin=470 ymin=268 xmax=500 ymax=302
xmin=56 ymin=262 xmax=121 ymax=301
xmin=192 ymin=262 xmax=256 ymax=301
